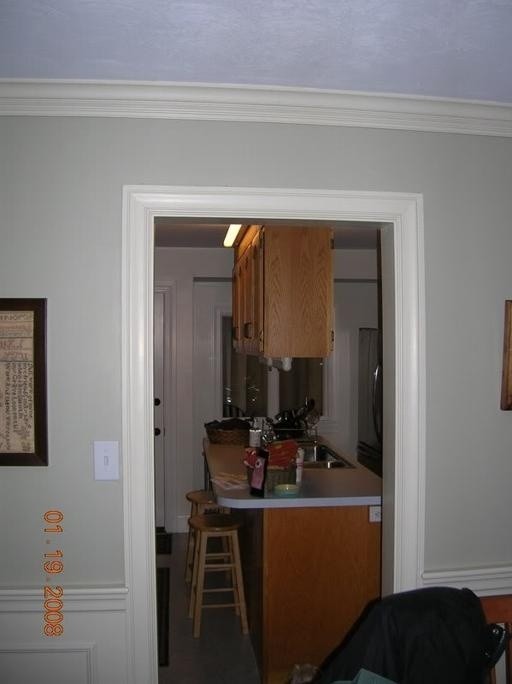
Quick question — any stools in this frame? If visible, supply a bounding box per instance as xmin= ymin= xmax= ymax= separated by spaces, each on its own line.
xmin=183 ymin=487 xmax=253 ymax=640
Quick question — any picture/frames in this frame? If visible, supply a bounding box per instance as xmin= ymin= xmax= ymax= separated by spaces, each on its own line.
xmin=0 ymin=297 xmax=49 ymax=468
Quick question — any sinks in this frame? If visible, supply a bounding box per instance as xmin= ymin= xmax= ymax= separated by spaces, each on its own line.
xmin=299 ymin=443 xmax=342 ymax=462
xmin=301 ymin=461 xmax=356 ymax=470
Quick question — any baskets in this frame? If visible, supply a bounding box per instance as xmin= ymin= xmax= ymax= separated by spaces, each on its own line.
xmin=204 ymin=423 xmax=249 ymax=444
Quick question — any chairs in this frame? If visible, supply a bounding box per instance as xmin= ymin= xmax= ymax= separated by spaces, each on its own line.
xmin=328 ymin=587 xmax=512 ymax=683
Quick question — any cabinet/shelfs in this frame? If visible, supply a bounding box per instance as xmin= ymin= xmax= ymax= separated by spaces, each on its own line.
xmin=227 ymin=220 xmax=337 ymax=360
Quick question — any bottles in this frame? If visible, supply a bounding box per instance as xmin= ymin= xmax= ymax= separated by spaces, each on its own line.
xmin=274 ymin=399 xmax=314 ymax=428
